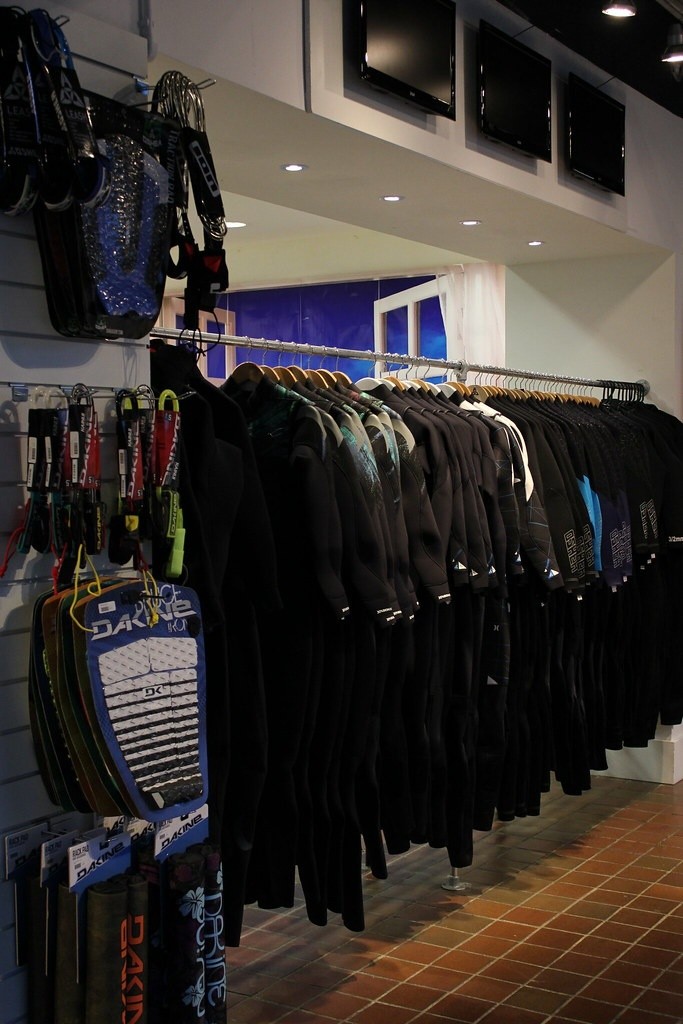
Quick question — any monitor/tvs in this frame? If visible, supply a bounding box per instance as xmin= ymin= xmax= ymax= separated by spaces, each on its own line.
xmin=348 ymin=0 xmax=457 ymax=122
xmin=565 ymin=72 xmax=625 ymax=198
xmin=476 ymin=19 xmax=552 ymax=164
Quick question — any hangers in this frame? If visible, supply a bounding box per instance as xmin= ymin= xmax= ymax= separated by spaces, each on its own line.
xmin=227 ymin=335 xmax=659 ymax=409
